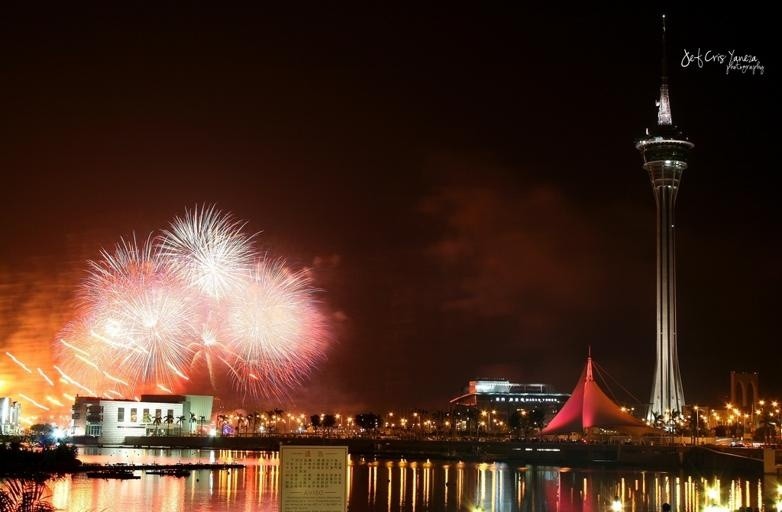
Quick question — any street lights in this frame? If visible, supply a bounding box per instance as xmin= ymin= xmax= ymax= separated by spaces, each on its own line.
xmin=666 ymin=401 xmax=781 ymax=447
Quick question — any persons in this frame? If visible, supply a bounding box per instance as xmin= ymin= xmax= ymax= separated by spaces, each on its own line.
xmin=662 ymin=502 xmax=670 ymax=512
xmin=38 ymin=432 xmax=45 ymax=446
